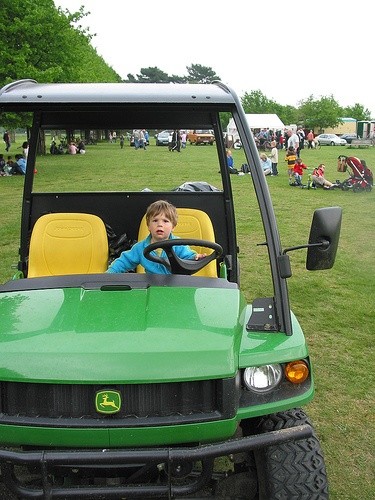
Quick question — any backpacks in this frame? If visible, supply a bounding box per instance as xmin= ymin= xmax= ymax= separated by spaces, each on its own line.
xmin=240 ymin=164 xmax=249 ymax=173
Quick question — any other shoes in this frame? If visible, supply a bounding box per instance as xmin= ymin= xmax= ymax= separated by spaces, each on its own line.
xmin=329 ymin=184 xmax=340 ymax=188
xmin=322 ymin=186 xmax=334 ymax=190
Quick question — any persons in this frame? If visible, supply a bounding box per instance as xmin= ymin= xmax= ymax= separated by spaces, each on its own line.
xmin=260 ymin=128 xmax=284 ymax=149
xmin=169 ymin=131 xmax=186 ymax=152
xmin=313 ymin=164 xmax=333 ymax=186
xmin=109 ymin=135 xmax=112 ymax=142
xmin=297 ymin=128 xmax=305 ymax=150
xmin=120 ymin=139 xmax=124 ymax=149
xmin=307 ymin=130 xmax=314 ymax=149
xmin=134 ymin=129 xmax=149 ymax=150
xmin=0 ymin=142 xmax=29 ymax=176
xmin=314 ymin=135 xmax=319 ymax=149
xmin=286 ymin=128 xmax=299 ymax=149
xmin=226 ymin=148 xmax=237 ymax=174
xmin=106 ymin=201 xmax=206 ymax=274
xmin=4 ymin=130 xmax=10 ymax=152
xmin=260 ymin=153 xmax=272 ymax=175
xmin=294 ymin=159 xmax=309 ymax=182
xmin=269 ymin=141 xmax=279 ymax=176
xmin=51 ymin=140 xmax=85 ymax=155
xmin=285 ymin=146 xmax=297 ymax=181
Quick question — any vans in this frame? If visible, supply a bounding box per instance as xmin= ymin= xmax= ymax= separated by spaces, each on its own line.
xmin=153 ymin=131 xmax=186 ymax=143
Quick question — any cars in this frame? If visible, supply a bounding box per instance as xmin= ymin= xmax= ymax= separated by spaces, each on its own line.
xmin=186 ymin=129 xmax=214 ymax=146
xmin=130 ymin=135 xmax=147 ymax=146
xmin=315 ymin=133 xmax=347 ymax=146
xmin=156 ymin=132 xmax=171 ymax=146
xmin=339 ymin=133 xmax=360 ymax=143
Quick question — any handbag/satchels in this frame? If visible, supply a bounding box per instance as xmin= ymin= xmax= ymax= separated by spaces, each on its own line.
xmin=338 ymin=159 xmax=348 ymax=172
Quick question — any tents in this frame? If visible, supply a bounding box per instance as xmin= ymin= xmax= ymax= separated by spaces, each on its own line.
xmin=227 ymin=114 xmax=285 ymax=137
